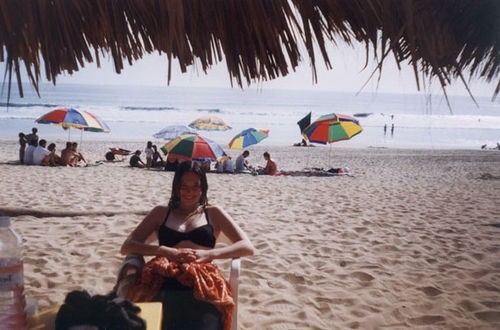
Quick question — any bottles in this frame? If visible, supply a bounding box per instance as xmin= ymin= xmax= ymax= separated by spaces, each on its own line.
xmin=0 ymin=216 xmax=27 ymax=330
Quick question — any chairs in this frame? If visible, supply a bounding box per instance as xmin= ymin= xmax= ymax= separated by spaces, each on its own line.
xmin=125 ymin=240 xmax=241 ymax=330
xmin=110 ymin=148 xmax=135 ymax=161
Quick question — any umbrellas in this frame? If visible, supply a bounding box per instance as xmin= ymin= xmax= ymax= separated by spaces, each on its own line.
xmin=303 ymin=113 xmax=364 ymax=169
xmin=151 ymin=125 xmax=199 ymax=140
xmin=187 ymin=115 xmax=233 ymax=132
xmin=159 ymin=132 xmax=232 ymax=166
xmin=228 ymin=127 xmax=270 ymax=150
xmin=33 ymin=103 xmax=111 ymax=142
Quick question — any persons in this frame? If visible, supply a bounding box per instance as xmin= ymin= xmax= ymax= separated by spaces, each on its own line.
xmin=129 ymin=141 xmax=211 ymax=171
xmin=19 ymin=128 xmax=90 ymax=167
xmin=120 ymin=160 xmax=256 ymax=330
xmin=215 ymin=151 xmax=277 ymax=176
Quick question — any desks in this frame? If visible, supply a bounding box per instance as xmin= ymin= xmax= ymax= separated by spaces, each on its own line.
xmin=24 ymin=302 xmax=162 ymax=330
